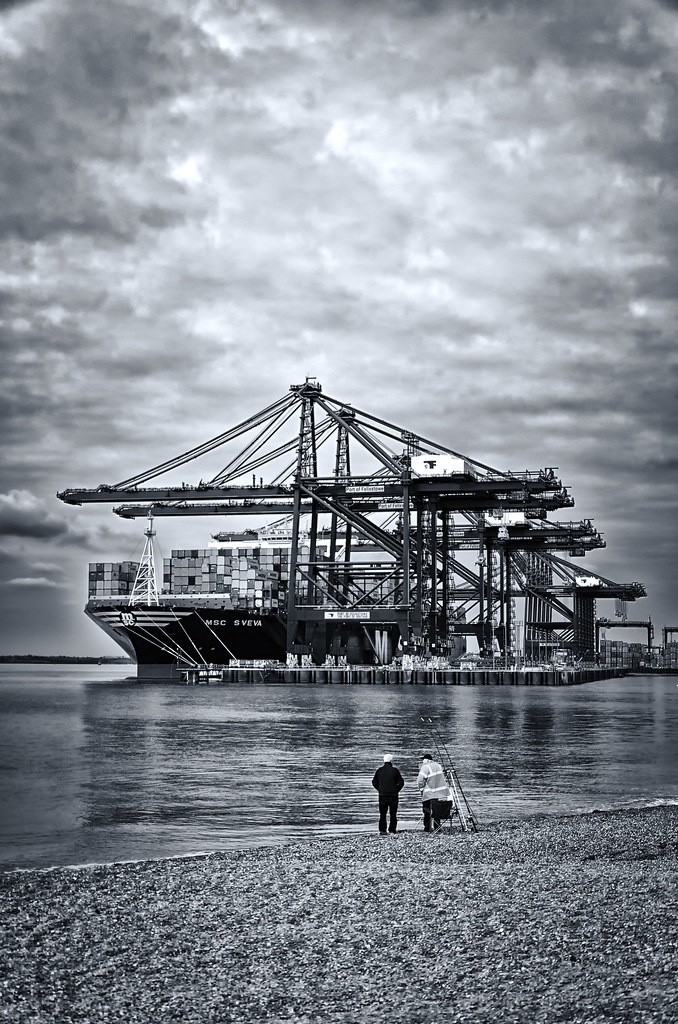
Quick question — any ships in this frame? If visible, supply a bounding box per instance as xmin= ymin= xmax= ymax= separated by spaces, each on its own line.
xmin=84 ymin=548 xmax=465 ymax=681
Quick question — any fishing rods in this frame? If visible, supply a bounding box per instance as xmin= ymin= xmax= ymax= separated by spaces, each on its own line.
xmin=426 ymin=716 xmax=481 ymax=831
xmin=417 ymin=715 xmax=471 ymax=833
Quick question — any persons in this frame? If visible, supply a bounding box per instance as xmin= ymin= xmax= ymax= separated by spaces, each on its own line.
xmin=417 ymin=754 xmax=450 ymax=833
xmin=372 ymin=754 xmax=404 ymax=835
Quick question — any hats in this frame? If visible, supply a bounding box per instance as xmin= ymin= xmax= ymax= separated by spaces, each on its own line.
xmin=420 ymin=754 xmax=433 ymax=760
xmin=384 ymin=754 xmax=393 ymax=763
